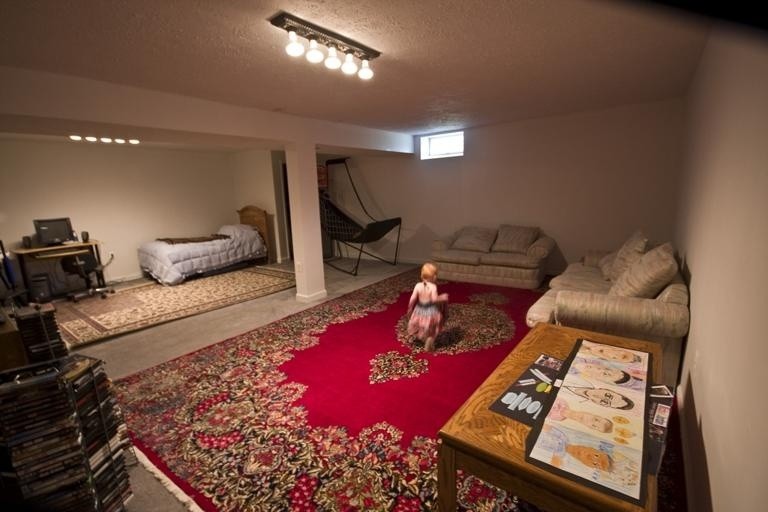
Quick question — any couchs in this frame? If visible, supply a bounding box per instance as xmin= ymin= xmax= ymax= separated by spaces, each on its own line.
xmin=527 ymin=228 xmax=690 ymax=397
xmin=431 ymin=224 xmax=555 ymax=290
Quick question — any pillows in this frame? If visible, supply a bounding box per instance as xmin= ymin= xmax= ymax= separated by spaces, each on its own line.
xmin=493 ymin=226 xmax=540 ymax=254
xmin=598 ymin=250 xmax=618 ymax=280
xmin=610 ymin=228 xmax=650 ymax=283
xmin=452 ymin=227 xmax=498 ymax=253
xmin=608 ymin=241 xmax=678 ymax=299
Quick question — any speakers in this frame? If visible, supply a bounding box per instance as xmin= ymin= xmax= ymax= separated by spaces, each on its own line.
xmin=22 ymin=236 xmax=32 ymax=248
xmin=82 ymin=231 xmax=89 ymax=243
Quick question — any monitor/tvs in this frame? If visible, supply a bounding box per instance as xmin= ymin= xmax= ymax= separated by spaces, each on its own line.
xmin=33 ymin=216 xmax=75 ymax=246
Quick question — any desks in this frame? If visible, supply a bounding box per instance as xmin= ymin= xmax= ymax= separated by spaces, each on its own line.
xmin=437 ymin=321 xmax=663 ymax=511
xmin=9 ymin=240 xmax=106 ymax=307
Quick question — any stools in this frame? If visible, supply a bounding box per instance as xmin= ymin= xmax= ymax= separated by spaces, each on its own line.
xmin=59 ymin=253 xmax=115 ymax=304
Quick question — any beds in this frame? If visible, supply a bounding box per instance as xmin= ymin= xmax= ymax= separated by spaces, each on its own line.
xmin=137 ymin=205 xmax=275 ymax=287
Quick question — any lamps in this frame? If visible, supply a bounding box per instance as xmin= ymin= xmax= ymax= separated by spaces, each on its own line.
xmin=266 ymin=10 xmax=382 ymax=82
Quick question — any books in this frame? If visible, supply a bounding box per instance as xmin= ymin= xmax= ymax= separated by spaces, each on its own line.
xmin=14 ymin=303 xmax=69 ymax=364
xmin=0 ymin=368 xmax=99 ymax=512
xmin=54 ymin=353 xmax=134 ymax=512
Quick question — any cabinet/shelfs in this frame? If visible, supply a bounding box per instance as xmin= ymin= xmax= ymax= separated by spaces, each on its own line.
xmin=0 ymin=354 xmax=135 ymax=512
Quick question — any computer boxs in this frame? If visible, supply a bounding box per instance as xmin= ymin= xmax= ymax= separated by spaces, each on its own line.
xmin=30 ymin=274 xmax=53 ymax=303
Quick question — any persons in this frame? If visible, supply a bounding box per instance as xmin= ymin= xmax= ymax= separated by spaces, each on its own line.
xmin=545 ymin=397 xmax=615 ymax=435
xmin=406 ymin=262 xmax=449 ymax=351
xmin=580 ymin=344 xmax=642 ymax=366
xmin=556 ymin=378 xmax=634 ymax=411
xmin=568 ymin=356 xmax=631 ymax=385
xmin=530 ymin=424 xmax=615 ymax=475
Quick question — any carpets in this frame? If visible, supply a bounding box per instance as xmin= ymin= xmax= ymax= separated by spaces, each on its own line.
xmin=109 ymin=251 xmax=530 ymax=512
xmin=1 ymin=266 xmax=296 ymax=351
